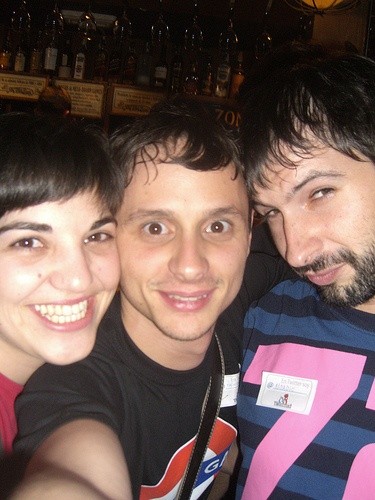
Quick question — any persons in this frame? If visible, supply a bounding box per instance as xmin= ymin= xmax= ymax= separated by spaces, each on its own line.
xmin=8 ymin=99 xmax=299 ymax=500
xmin=234 ymin=44 xmax=375 ymax=500
xmin=0 ymin=112 xmax=121 ymax=455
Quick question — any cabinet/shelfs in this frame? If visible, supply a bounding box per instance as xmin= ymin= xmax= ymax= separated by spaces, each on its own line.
xmin=0 ymin=1 xmax=246 ymax=125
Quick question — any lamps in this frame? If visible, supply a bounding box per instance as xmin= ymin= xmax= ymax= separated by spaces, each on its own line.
xmin=284 ymin=0 xmax=360 ymax=16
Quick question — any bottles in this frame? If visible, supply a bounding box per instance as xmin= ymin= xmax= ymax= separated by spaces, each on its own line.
xmin=0 ymin=0 xmax=311 ymax=98
